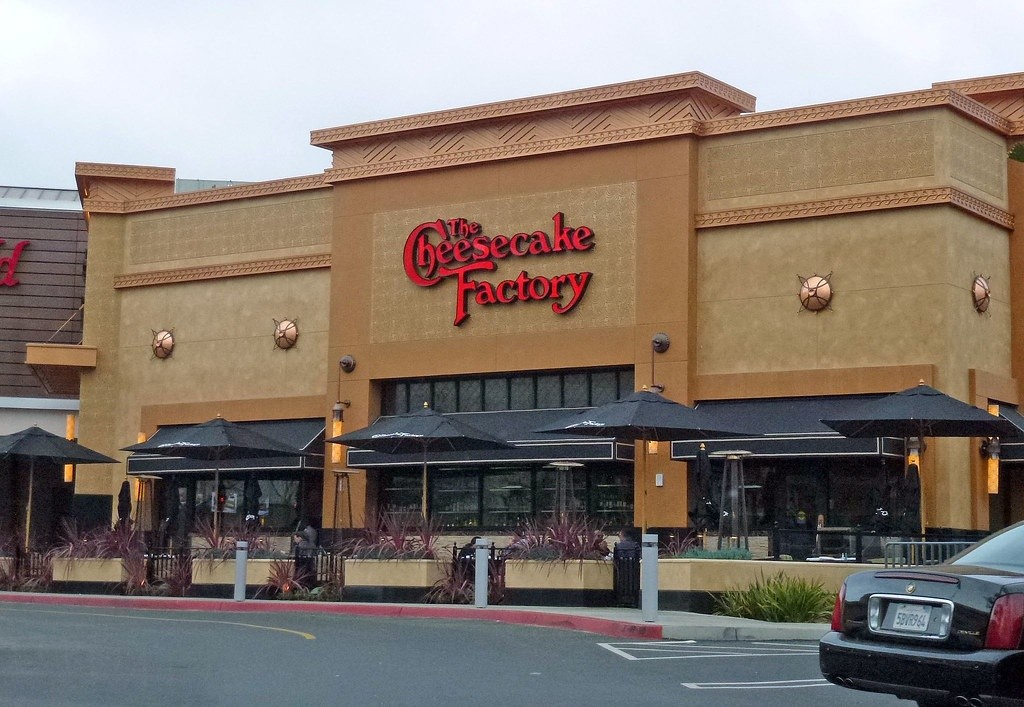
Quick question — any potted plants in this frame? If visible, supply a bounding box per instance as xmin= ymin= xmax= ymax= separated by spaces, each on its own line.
xmin=639 ymin=529 xmax=925 ymax=594
xmin=1 ymin=499 xmax=620 ymax=612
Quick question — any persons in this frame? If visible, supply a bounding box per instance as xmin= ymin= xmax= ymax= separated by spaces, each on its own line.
xmin=454 ymin=527 xmax=639 ymax=563
xmin=288 ymin=532 xmax=317 ymax=562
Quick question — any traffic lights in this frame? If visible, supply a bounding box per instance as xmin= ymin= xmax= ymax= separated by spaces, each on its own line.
xmin=219 ymin=495 xmax=227 ymax=511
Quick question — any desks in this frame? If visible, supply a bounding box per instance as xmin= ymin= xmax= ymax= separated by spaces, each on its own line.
xmin=462 ymin=558 xmax=501 ymax=579
xmin=806 ymin=558 xmax=856 ymax=563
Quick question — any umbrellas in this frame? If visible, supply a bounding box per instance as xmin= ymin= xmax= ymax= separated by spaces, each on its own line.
xmin=0 ymin=422 xmax=121 ymax=555
xmin=819 ymin=375 xmax=1012 ymax=483
xmin=118 ymin=476 xmax=134 ymax=524
xmin=119 ymin=416 xmax=315 ymax=548
xmin=324 ymin=403 xmax=519 ymax=546
xmin=530 ymin=385 xmax=768 ymax=548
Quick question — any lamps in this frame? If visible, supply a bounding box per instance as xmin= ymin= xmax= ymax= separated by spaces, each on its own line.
xmin=649 ymin=333 xmax=670 ymax=454
xmin=332 ymin=355 xmax=356 ymax=464
xmin=907 ymin=437 xmax=926 ymax=466
xmin=979 ymin=405 xmax=1001 ymax=494
xmin=65 ymin=415 xmax=78 ymax=482
xmin=135 ymin=432 xmax=146 ymax=502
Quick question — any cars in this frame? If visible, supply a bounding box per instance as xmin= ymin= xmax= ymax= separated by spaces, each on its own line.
xmin=819 ymin=521 xmax=1023 ymax=707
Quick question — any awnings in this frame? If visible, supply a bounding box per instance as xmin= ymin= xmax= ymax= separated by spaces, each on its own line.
xmin=997 ymin=406 xmax=1024 ymax=462
xmin=344 ymin=400 xmax=636 ymax=470
xmin=669 ymin=392 xmax=908 ymax=460
xmin=117 ymin=416 xmax=326 ymax=481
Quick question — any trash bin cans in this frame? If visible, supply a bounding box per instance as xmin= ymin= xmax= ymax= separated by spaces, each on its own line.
xmin=295 ymin=547 xmax=317 ymax=589
xmin=612 ymin=545 xmax=640 ymax=609
xmin=452 ymin=542 xmax=506 ymax=604
